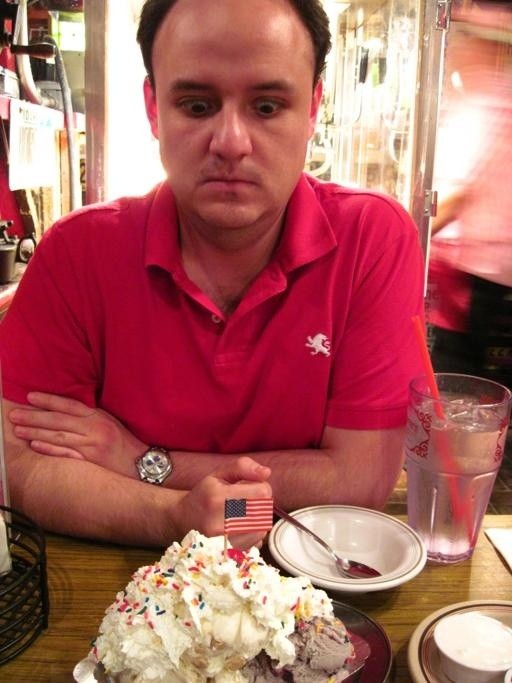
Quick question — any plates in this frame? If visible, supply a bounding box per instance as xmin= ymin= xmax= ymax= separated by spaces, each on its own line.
xmin=72 ymin=593 xmax=393 ymax=682
xmin=504 ymin=668 xmax=510 ymax=683
xmin=268 ymin=503 xmax=427 ymax=591
xmin=408 ymin=599 xmax=511 ymax=683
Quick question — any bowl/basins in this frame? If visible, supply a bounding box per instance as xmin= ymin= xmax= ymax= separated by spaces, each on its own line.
xmin=432 ymin=612 xmax=511 ymax=682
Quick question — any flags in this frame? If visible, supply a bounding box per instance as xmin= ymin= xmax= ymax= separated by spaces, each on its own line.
xmin=224 ymin=497 xmax=275 ymax=535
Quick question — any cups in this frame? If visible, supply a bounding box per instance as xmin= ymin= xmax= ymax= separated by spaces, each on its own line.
xmin=407 ymin=370 xmax=511 ymax=565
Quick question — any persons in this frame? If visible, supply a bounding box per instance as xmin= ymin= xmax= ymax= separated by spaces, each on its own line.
xmin=0 ymin=0 xmax=433 ymax=552
xmin=430 ymin=151 xmax=512 ymax=385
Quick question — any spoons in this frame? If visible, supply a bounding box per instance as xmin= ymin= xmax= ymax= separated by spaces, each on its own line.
xmin=268 ymin=502 xmax=384 ymax=578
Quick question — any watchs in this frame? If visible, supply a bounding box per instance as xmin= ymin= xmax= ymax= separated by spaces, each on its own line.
xmin=134 ymin=448 xmax=174 ymax=485
